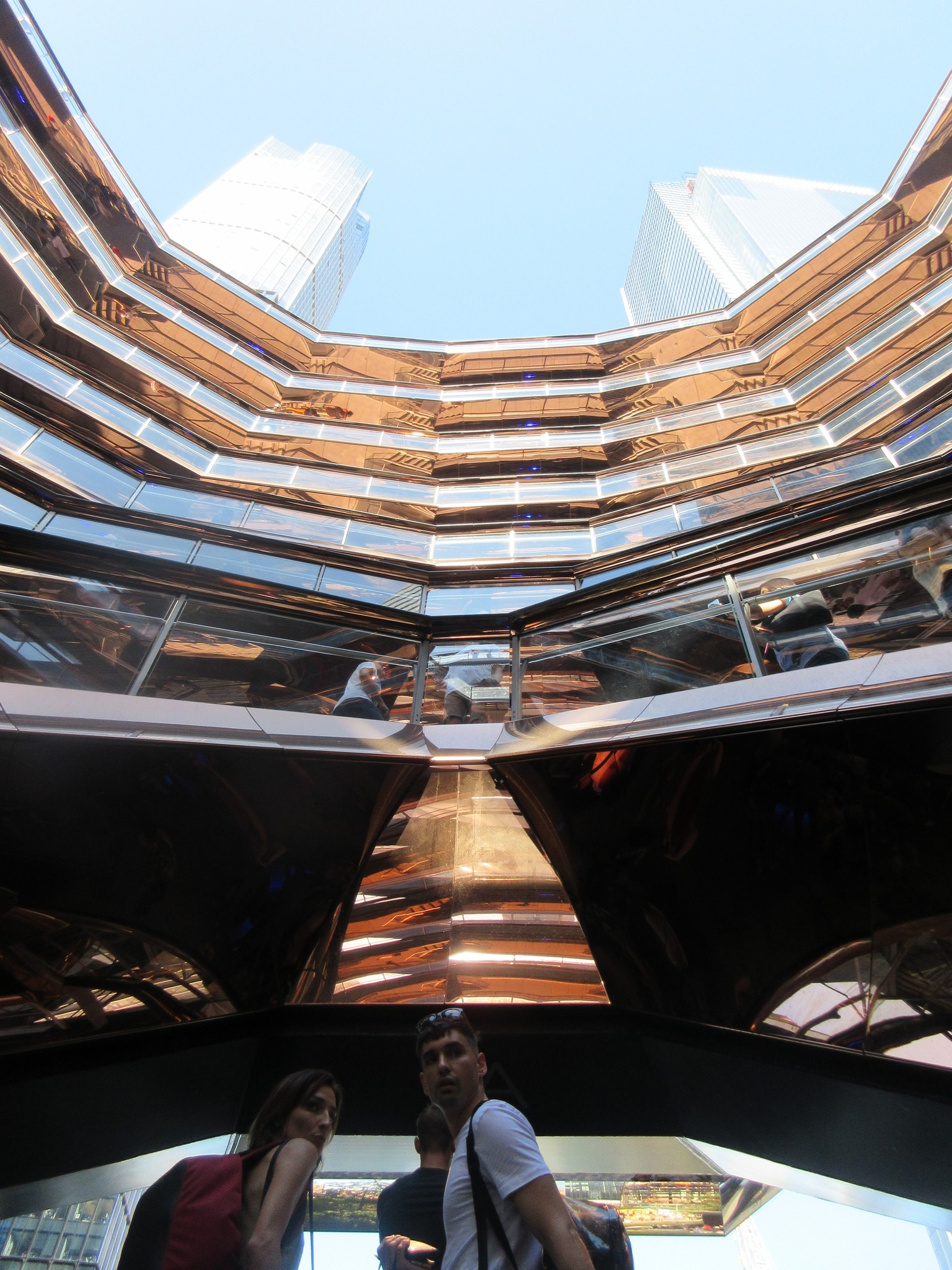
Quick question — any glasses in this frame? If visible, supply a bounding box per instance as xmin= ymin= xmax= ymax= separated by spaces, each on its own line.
xmin=416 ymin=1008 xmax=470 ymax=1032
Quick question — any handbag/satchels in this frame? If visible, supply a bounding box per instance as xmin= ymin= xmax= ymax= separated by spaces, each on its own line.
xmin=766 ymin=588 xmax=834 ymax=634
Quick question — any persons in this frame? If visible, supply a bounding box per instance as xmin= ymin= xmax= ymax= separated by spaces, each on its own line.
xmin=444 ymin=643 xmax=505 ymax=725
xmin=236 ymin=1066 xmax=341 ymax=1270
xmin=708 ymin=578 xmax=850 ymax=673
xmin=282 ymin=391 xmax=349 ymax=420
xmin=377 ymin=1102 xmax=455 ymax=1270
xmin=113 ymin=298 xmax=138 ymax=320
xmin=333 ymin=657 xmax=390 ymax=721
xmin=860 ymin=517 xmax=952 ymax=653
xmin=833 ymin=569 xmax=910 ymax=620
xmin=50 ymin=229 xmax=80 ymax=275
xmin=377 ymin=1008 xmax=595 ymax=1270
xmin=90 ymin=185 xmax=113 ymax=219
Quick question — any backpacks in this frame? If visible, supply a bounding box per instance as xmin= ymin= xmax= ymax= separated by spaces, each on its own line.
xmin=466 ymin=1099 xmax=635 ymax=1270
xmin=116 ymin=1136 xmax=306 ymax=1270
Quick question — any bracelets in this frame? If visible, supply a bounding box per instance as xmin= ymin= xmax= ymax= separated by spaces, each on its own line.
xmin=895 ymin=548 xmax=904 ymax=559
xmin=378 ymin=699 xmax=384 ymax=707
